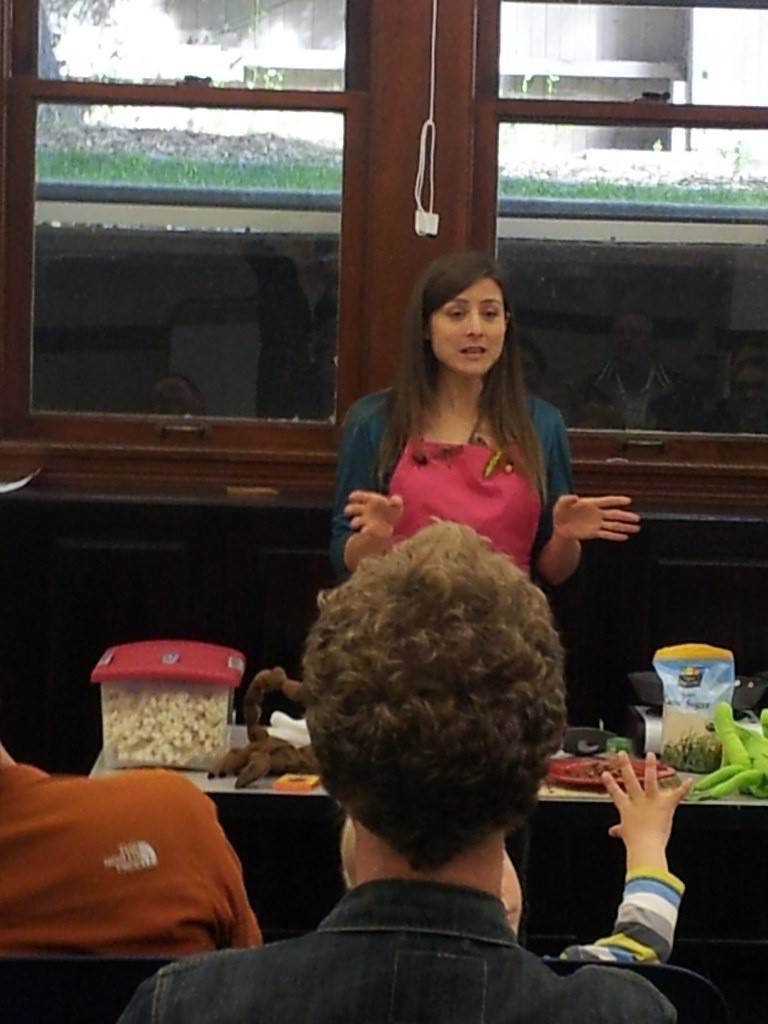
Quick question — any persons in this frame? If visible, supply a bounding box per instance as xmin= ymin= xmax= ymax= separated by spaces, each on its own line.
xmin=119 ymin=520 xmax=678 ymax=1024
xmin=0 ymin=743 xmax=262 ymax=959
xmin=332 ymin=253 xmax=641 ymax=587
xmin=342 ymin=751 xmax=694 ymax=965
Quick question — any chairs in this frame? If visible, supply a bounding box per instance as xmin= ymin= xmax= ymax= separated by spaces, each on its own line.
xmin=0 ymin=956 xmax=177 ymax=1024
xmin=541 ymin=957 xmax=735 ymax=1024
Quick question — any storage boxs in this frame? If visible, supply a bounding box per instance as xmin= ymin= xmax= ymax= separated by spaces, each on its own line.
xmin=91 ymin=639 xmax=246 ymax=772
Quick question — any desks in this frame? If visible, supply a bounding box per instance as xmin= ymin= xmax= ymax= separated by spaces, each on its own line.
xmin=86 ymin=726 xmax=768 ymax=984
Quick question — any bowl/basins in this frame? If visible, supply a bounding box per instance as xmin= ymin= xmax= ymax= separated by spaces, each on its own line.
xmin=632 ymin=670 xmax=768 ymax=718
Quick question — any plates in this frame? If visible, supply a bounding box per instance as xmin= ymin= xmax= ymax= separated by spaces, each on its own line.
xmin=547 ymin=756 xmax=674 ymax=787
